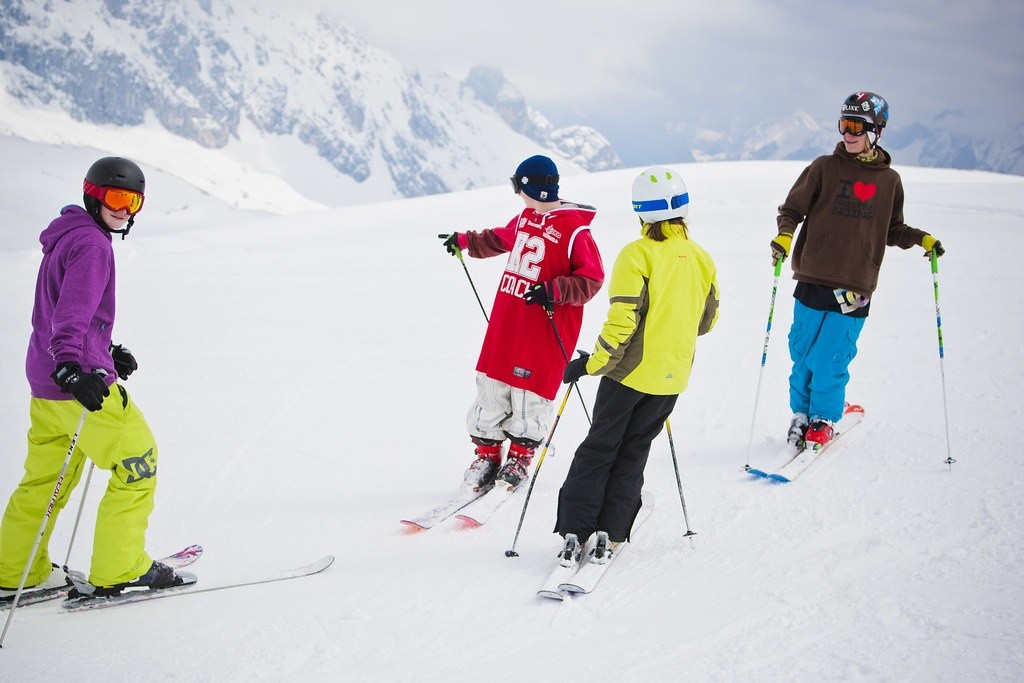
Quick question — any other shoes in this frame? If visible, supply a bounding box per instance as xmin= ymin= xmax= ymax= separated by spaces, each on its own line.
xmin=94 ymin=559 xmax=175 ymax=586
xmin=787 ymin=413 xmax=809 ymax=446
xmin=559 ymin=540 xmax=585 ymax=561
xmin=806 ymin=416 xmax=834 ymax=445
xmin=0 ymin=563 xmax=73 ymax=602
xmin=588 ymin=531 xmax=621 ymax=560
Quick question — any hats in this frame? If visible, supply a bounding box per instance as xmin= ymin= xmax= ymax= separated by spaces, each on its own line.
xmin=516 ymin=155 xmax=559 ymax=202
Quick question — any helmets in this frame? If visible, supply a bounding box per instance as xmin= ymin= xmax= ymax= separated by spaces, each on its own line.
xmin=840 ymin=91 xmax=889 ymax=127
xmin=632 ymin=168 xmax=689 ymax=224
xmin=84 ymin=157 xmax=145 ymax=219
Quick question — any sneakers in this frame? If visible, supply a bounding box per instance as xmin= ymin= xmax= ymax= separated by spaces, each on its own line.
xmin=463 ymin=444 xmax=501 ymax=487
xmin=498 ymin=444 xmax=533 ymax=486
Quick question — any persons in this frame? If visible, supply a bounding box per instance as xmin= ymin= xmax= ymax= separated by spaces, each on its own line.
xmin=766 ymin=91 xmax=946 ymax=452
xmin=439 ymin=154 xmax=607 ymax=493
xmin=0 ymin=157 xmax=184 ymax=600
xmin=552 ymin=166 xmax=719 ymax=569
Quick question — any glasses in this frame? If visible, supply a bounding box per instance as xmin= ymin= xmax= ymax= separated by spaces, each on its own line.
xmin=838 ymin=117 xmax=867 ymax=136
xmin=99 ymin=186 xmax=145 ymax=215
xmin=509 ymin=175 xmax=519 ymax=193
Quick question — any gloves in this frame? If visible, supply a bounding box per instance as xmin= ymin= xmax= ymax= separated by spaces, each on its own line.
xmin=50 ymin=361 xmax=109 ymax=412
xmin=108 ymin=340 xmax=137 ymax=380
xmin=523 ymin=281 xmax=559 ymax=306
xmin=438 ymin=232 xmax=468 ymax=256
xmin=921 ymin=234 xmax=945 ymax=261
xmin=770 ymin=232 xmax=794 ymax=267
xmin=563 ymin=355 xmax=588 ymax=383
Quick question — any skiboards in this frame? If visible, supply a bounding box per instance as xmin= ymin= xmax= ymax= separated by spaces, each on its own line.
xmin=399 ymin=475 xmax=529 ymax=530
xmin=1 ymin=542 xmax=338 ymax=614
xmin=535 ymin=490 xmax=657 ymax=601
xmin=745 ymin=400 xmax=866 ymax=483
